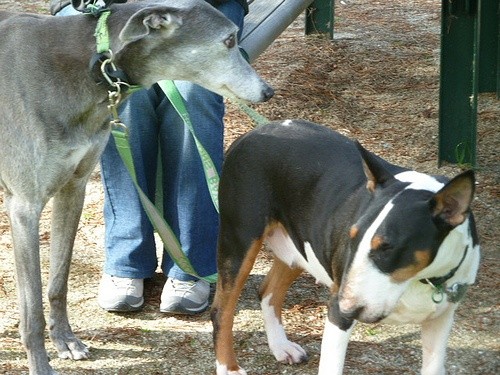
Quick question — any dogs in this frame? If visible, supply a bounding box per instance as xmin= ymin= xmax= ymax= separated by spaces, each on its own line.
xmin=209 ymin=118 xmax=482 ymax=375
xmin=0 ymin=1 xmax=275 ymax=375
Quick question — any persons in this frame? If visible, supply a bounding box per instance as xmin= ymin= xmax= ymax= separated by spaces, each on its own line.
xmin=52 ymin=0 xmax=249 ymax=316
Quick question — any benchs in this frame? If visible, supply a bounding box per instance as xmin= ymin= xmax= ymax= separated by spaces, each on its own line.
xmin=52 ymin=0 xmax=334 ymax=64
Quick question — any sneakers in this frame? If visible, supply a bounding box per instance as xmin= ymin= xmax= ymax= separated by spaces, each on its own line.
xmin=160 ymin=277 xmax=210 ymax=315
xmin=98 ymin=271 xmax=144 ymax=311
